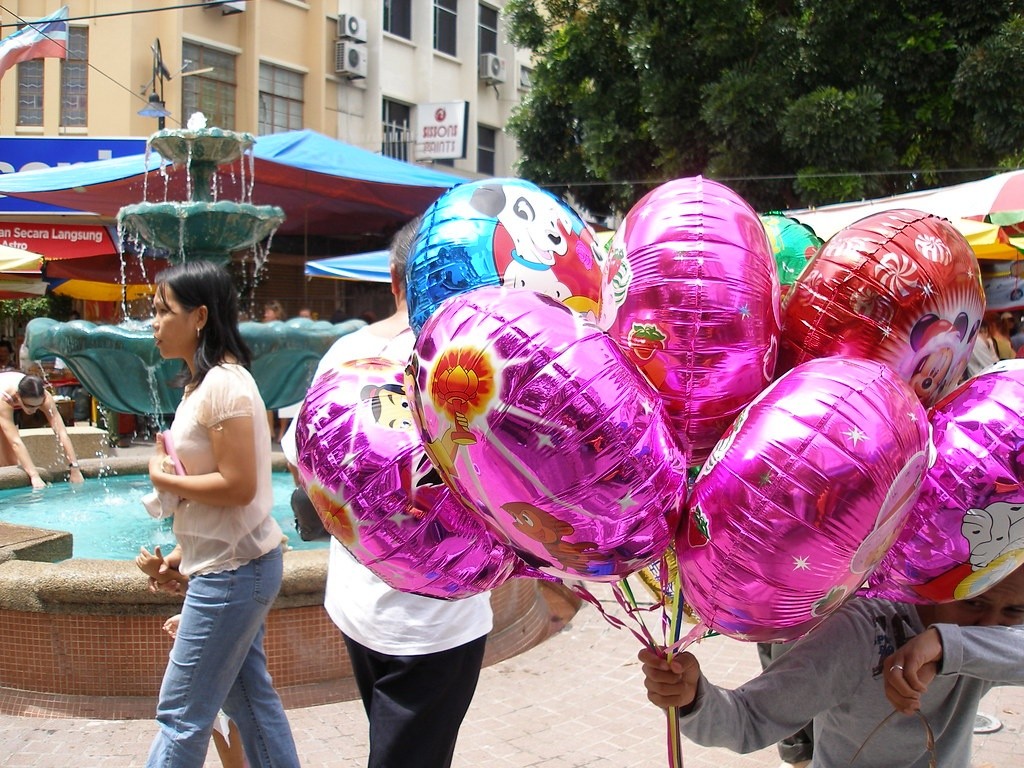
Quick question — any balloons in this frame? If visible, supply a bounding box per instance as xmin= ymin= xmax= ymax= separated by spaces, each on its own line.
xmin=294 ymin=174 xmax=1024 ymax=643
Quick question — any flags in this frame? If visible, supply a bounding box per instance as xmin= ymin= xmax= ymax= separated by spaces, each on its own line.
xmin=0 ymin=6 xmax=68 ymax=81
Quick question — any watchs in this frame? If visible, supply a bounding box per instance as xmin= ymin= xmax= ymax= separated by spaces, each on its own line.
xmin=69 ymin=463 xmax=78 ymax=467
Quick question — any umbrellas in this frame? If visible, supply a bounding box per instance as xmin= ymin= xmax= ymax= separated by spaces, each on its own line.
xmin=303 ymin=247 xmax=394 ymax=282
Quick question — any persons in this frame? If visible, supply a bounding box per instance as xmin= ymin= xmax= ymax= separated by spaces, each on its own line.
xmin=280 ymin=215 xmax=493 ymax=768
xmin=983 ymin=311 xmax=1016 ymax=360
xmin=1001 ymin=312 xmax=1015 ymax=328
xmin=1011 ymin=321 xmax=1024 ymax=352
xmin=967 ymin=321 xmax=994 ymax=377
xmin=0 ymin=372 xmax=85 ymax=488
xmin=136 ymin=261 xmax=302 ymax=768
xmin=0 ymin=340 xmax=16 ymax=369
xmin=638 ymin=581 xmax=1024 ymax=768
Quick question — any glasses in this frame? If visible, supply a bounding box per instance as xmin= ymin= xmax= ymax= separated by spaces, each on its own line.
xmin=18 ymin=391 xmax=46 ymax=409
xmin=849 ymin=708 xmax=936 ymax=768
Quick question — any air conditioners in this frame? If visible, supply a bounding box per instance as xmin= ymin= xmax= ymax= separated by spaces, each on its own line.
xmin=479 ymin=53 xmax=506 ymax=84
xmin=336 ymin=13 xmax=367 ymax=44
xmin=335 ymin=42 xmax=368 ymax=80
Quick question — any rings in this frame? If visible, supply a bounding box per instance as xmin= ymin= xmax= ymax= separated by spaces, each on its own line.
xmin=890 ymin=665 xmax=904 ymax=671
